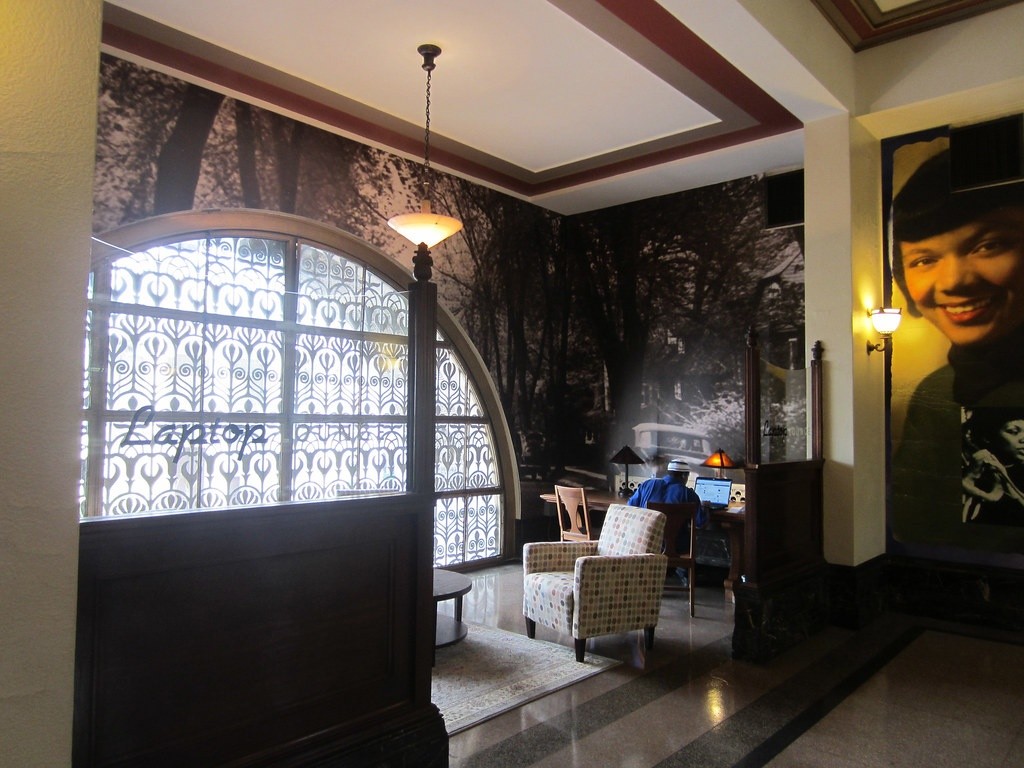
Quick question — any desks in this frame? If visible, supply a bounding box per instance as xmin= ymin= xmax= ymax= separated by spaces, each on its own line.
xmin=540 ymin=490 xmax=747 ymax=603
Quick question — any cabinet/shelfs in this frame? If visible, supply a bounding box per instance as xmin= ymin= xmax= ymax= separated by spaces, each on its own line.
xmin=433 ymin=567 xmax=472 ymax=667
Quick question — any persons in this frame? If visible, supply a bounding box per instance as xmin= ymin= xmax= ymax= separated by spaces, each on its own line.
xmin=627 ymin=459 xmax=711 ymax=587
xmin=888 ymin=140 xmax=1024 ymax=629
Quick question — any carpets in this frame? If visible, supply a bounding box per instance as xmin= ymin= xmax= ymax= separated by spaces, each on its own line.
xmin=430 ymin=620 xmax=624 ymax=737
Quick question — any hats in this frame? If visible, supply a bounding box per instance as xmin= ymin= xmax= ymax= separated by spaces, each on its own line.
xmin=668 ymin=458 xmax=690 ymax=472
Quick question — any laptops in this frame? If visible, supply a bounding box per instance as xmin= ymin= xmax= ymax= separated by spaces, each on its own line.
xmin=694 ymin=477 xmax=733 ymax=511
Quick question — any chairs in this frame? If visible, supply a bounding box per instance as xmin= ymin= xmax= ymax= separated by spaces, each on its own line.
xmin=522 ymin=504 xmax=668 ymax=661
xmin=645 ymin=500 xmax=695 ymax=618
xmin=555 ymin=484 xmax=601 ymax=542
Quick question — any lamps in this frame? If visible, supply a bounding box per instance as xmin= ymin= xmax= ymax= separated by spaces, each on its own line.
xmin=699 ymin=448 xmax=740 ymax=478
xmin=387 ymin=44 xmax=463 ymax=249
xmin=866 ymin=306 xmax=903 ymax=356
xmin=608 ymin=445 xmax=645 ymax=496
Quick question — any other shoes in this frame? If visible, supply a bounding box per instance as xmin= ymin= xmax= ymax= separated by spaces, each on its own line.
xmin=673 ymin=571 xmax=687 ymax=591
